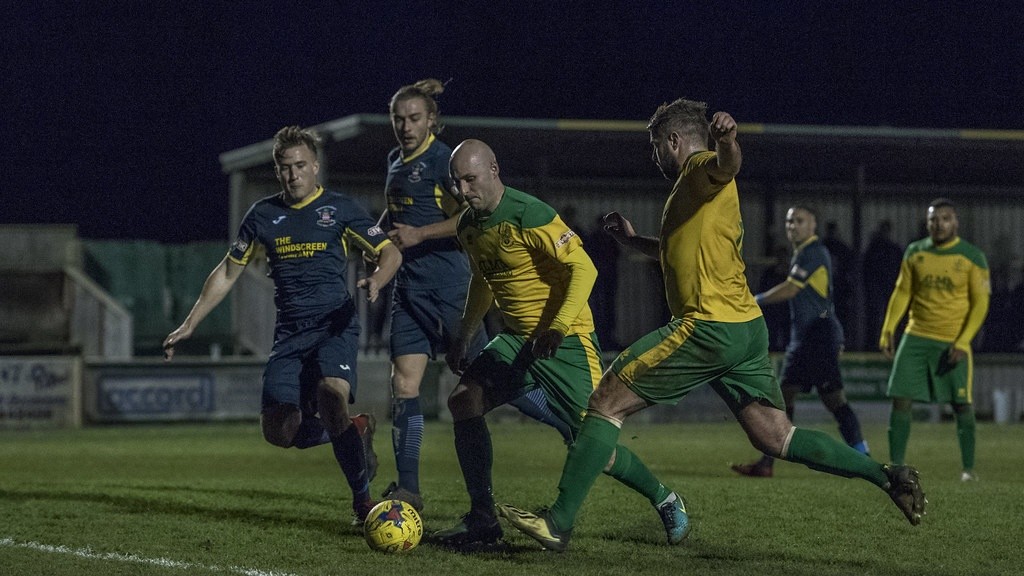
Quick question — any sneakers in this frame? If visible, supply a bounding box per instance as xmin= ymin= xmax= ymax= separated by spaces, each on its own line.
xmin=881 ymin=463 xmax=928 ymax=526
xmin=356 ymin=413 xmax=379 ymax=483
xmin=732 ymin=462 xmax=772 ymax=477
xmin=350 ymin=505 xmax=372 ymax=526
xmin=496 ymin=502 xmax=575 ymax=553
xmin=655 ymin=493 xmax=689 ymax=544
xmin=433 ymin=511 xmax=504 ymax=545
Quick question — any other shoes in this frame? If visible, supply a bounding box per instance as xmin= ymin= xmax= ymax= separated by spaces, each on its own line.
xmin=386 ymin=488 xmax=424 ymax=512
xmin=962 ymin=471 xmax=976 ymax=483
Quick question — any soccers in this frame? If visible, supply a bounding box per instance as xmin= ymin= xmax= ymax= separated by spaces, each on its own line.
xmin=362 ymin=498 xmax=424 ymax=556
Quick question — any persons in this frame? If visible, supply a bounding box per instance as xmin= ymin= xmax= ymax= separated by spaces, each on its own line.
xmin=160 ymin=126 xmax=403 ymax=528
xmin=366 ymin=77 xmax=573 ymax=515
xmin=495 ymin=98 xmax=928 ymax=554
xmin=428 ymin=138 xmax=693 ymax=547
xmin=877 ymin=198 xmax=991 ymax=483
xmin=725 ymin=201 xmax=872 ymax=478
xmin=550 ymin=202 xmax=1023 ymax=360
